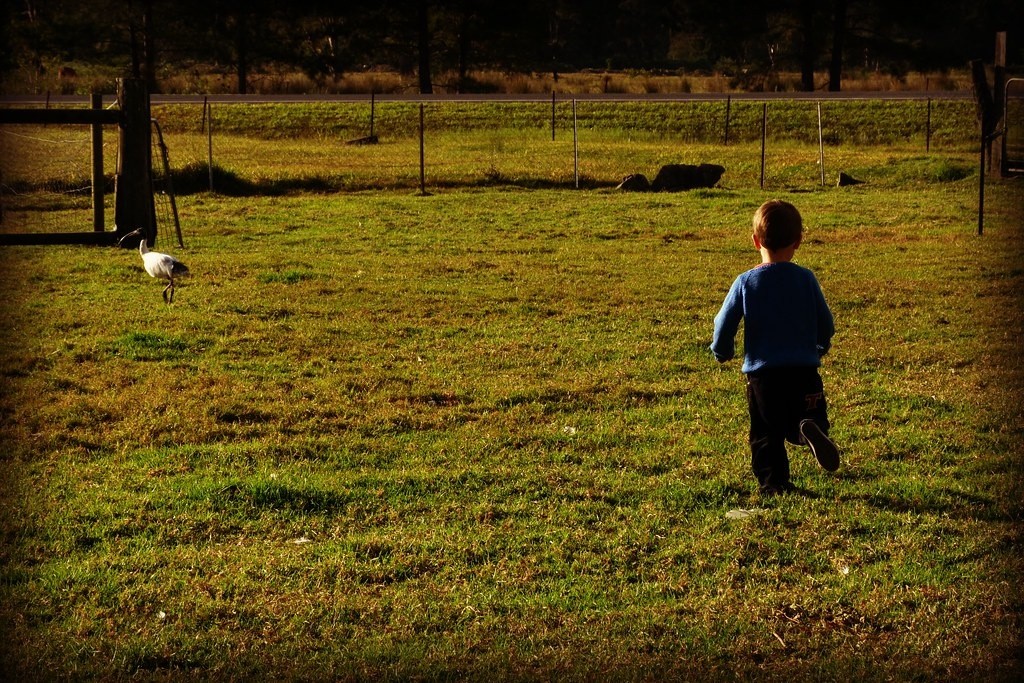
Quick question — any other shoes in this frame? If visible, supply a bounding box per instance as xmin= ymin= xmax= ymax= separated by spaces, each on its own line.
xmin=801 ymin=422 xmax=840 ymax=473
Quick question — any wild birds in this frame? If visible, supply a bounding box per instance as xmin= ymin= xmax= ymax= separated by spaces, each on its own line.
xmin=118 ymin=228 xmax=190 ymax=306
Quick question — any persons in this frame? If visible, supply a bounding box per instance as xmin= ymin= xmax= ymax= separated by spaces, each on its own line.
xmin=709 ymin=199 xmax=840 ymax=499
